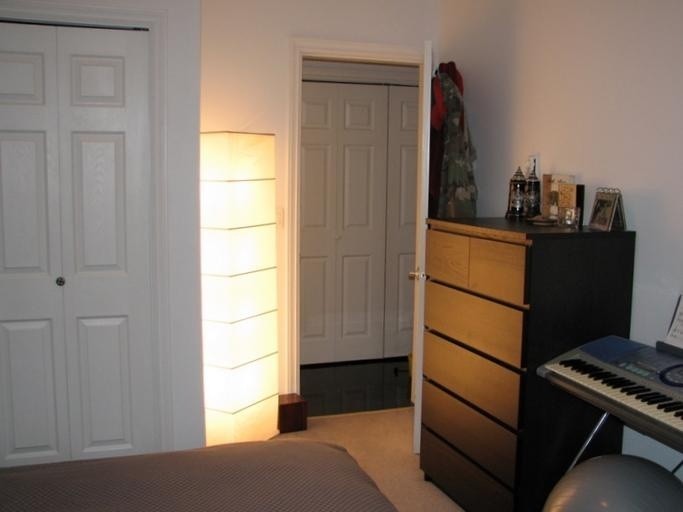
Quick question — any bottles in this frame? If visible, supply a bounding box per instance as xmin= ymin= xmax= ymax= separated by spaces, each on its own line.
xmin=524 ymin=158 xmax=540 ymax=217
xmin=508 ymin=166 xmax=527 ymax=216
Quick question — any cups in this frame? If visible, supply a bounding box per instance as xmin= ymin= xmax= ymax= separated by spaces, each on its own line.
xmin=557 ymin=206 xmax=581 ymax=233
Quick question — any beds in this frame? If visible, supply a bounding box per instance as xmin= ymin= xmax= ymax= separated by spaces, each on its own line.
xmin=1 ymin=439 xmax=398 ymax=512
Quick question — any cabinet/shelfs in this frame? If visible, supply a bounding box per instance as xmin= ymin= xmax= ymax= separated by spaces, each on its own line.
xmin=419 ymin=215 xmax=637 ymax=512
xmin=2 ymin=1 xmax=202 ymax=469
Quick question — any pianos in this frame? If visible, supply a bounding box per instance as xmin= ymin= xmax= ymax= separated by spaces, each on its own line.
xmin=535 ymin=334 xmax=683 ymax=452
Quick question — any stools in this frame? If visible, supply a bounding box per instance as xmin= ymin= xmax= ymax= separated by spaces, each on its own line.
xmin=543 ymin=455 xmax=682 ymax=512
